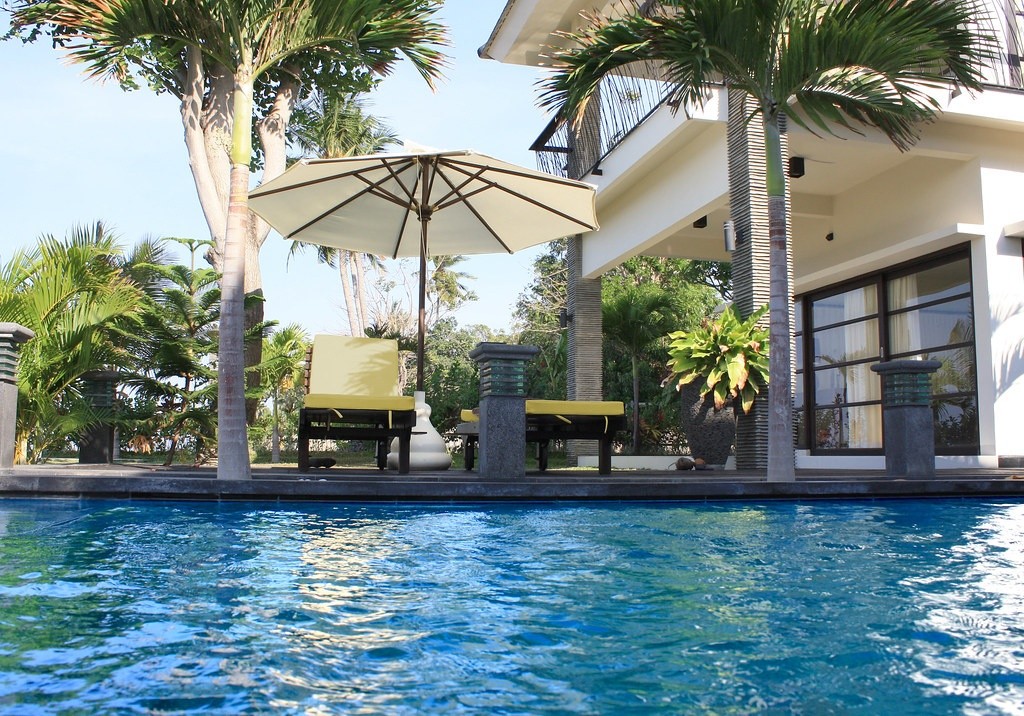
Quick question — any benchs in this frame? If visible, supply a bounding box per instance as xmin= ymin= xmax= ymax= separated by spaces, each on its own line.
xmin=455 ymin=400 xmax=627 ymax=474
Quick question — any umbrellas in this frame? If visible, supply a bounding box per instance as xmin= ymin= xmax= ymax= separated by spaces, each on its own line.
xmin=247 ymin=146 xmax=600 ymax=390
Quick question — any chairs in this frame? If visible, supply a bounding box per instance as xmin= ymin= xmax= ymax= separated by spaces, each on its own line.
xmin=298 ymin=334 xmax=417 ymax=475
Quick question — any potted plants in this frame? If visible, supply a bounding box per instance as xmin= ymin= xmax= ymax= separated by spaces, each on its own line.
xmin=661 ymin=302 xmax=770 ymax=470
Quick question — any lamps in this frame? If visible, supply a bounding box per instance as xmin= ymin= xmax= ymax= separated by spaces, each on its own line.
xmin=723 ymin=221 xmax=743 ymax=251
xmin=560 ymin=308 xmax=573 ymax=328
xmin=693 ymin=215 xmax=707 ymax=229
xmin=789 ymin=156 xmax=805 ymax=178
